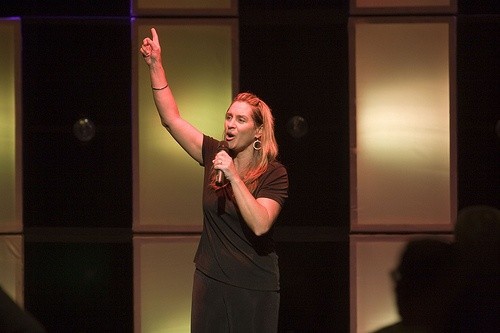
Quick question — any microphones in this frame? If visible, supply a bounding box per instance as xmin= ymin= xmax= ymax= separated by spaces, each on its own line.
xmin=215 ymin=140 xmax=229 ymax=186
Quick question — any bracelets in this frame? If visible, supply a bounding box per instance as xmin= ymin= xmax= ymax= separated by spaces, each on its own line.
xmin=151 ymin=84 xmax=168 ymax=90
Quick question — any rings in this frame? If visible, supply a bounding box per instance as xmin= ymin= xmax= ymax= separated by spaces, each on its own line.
xmin=220 ymin=160 xmax=222 ymax=164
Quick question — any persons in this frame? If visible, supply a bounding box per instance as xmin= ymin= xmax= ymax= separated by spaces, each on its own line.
xmin=139 ymin=26 xmax=290 ymax=333
xmin=368 ymin=234 xmax=499 ymax=333
xmin=453 ymin=203 xmax=500 ymax=333
